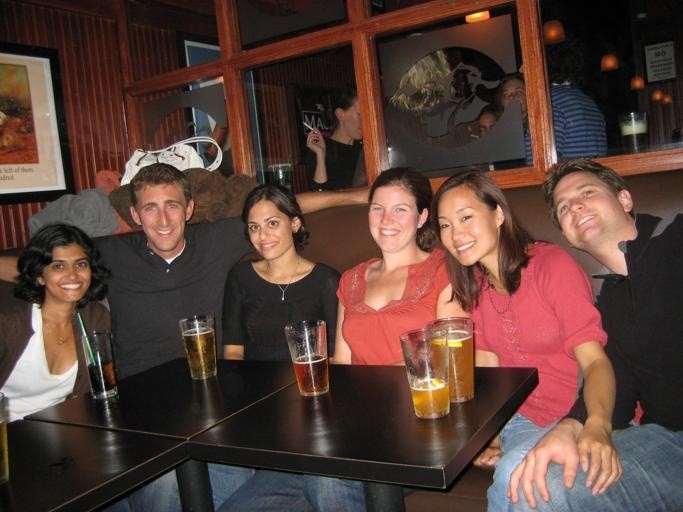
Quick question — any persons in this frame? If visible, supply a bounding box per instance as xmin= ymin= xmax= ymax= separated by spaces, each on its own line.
xmin=0 ymin=223 xmax=131 ymax=512
xmin=429 ymin=171 xmax=623 ymax=512
xmin=306 ymin=83 xmax=368 ymax=191
xmin=507 ymin=158 xmax=683 ymax=512
xmin=478 ymin=52 xmax=608 ymax=171
xmin=0 ymin=162 xmax=371 ymax=512
xmin=216 ymin=172 xmax=468 ymax=512
xmin=221 ymin=182 xmax=342 ymax=362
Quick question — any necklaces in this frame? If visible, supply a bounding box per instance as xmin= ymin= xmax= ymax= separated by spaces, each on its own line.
xmin=266 ymin=254 xmax=299 ymax=301
xmin=487 ymin=271 xmax=511 ymax=314
xmin=41 ymin=309 xmax=73 ymax=345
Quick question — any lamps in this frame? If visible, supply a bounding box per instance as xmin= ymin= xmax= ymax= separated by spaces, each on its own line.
xmin=446 ymin=7 xmax=678 ymax=105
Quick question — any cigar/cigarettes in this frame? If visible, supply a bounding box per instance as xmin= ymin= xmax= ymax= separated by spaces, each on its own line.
xmin=302 ymin=122 xmax=317 ymax=134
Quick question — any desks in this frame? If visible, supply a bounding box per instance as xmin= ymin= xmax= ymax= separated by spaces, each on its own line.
xmin=1 ymin=357 xmax=539 ymax=508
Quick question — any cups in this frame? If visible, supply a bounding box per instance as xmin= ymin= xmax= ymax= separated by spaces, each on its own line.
xmin=268 ymin=162 xmax=293 ymax=193
xmin=0 ymin=391 xmax=9 ymax=479
xmin=78 ymin=327 xmax=118 ymax=400
xmin=618 ymin=108 xmax=648 ymax=152
xmin=180 ymin=312 xmax=219 ymax=380
xmin=284 ymin=320 xmax=329 ymax=398
xmin=399 ymin=317 xmax=475 ymax=420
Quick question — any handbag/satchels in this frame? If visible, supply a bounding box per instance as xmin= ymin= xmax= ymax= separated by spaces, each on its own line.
xmin=118 ymin=135 xmax=223 ymax=187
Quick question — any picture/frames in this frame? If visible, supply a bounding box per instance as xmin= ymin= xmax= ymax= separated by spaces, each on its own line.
xmin=1 ymin=43 xmax=75 ymax=204
xmin=182 ymin=31 xmax=265 ymax=189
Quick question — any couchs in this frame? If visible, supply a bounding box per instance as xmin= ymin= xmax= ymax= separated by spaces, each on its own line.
xmin=1 ymin=149 xmax=683 ymax=508
